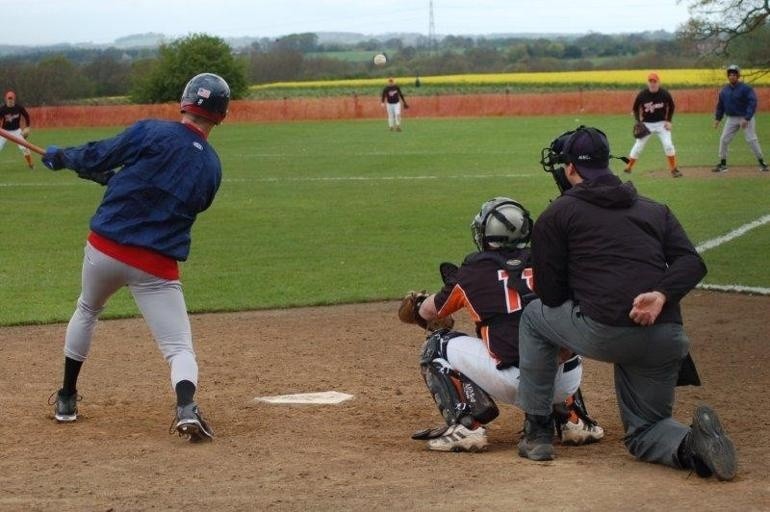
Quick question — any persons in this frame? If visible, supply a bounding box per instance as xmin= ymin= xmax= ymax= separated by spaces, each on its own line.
xmin=516 ymin=124 xmax=737 ymax=481
xmin=711 ymin=65 xmax=769 ymax=174
xmin=380 ymin=78 xmax=410 ymax=132
xmin=624 ymin=74 xmax=682 ymax=178
xmin=397 ymin=197 xmax=605 ymax=453
xmin=0 ymin=91 xmax=35 ymax=168
xmin=41 ymin=73 xmax=232 ymax=442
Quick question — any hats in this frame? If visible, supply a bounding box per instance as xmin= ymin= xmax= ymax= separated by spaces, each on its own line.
xmin=648 ymin=73 xmax=659 ymax=83
xmin=5 ymin=91 xmax=16 ymax=100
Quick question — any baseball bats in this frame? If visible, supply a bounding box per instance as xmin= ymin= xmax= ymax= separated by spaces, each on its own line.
xmin=0 ymin=128 xmax=46 ymax=155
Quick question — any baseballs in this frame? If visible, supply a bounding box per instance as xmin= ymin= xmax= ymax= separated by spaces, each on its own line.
xmin=374 ymin=55 xmax=385 ymax=65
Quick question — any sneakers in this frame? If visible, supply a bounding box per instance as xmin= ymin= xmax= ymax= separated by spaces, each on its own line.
xmin=685 ymin=406 xmax=735 ymax=481
xmin=517 ymin=414 xmax=555 ymax=462
xmin=561 ymin=417 xmax=605 ymax=446
xmin=759 ymin=162 xmax=769 ymax=172
xmin=48 ymin=389 xmax=83 ymax=422
xmin=169 ymin=403 xmax=213 ymax=443
xmin=426 ymin=424 xmax=490 ymax=453
xmin=711 ymin=164 xmax=728 ymax=173
xmin=672 ymin=168 xmax=682 ymax=178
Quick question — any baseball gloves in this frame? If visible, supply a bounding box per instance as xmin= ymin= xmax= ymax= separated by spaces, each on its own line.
xmin=633 ymin=122 xmax=650 ymax=138
xmin=399 ymin=290 xmax=454 ymax=332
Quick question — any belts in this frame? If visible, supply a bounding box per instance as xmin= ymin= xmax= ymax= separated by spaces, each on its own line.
xmin=564 ymin=358 xmax=579 ymax=372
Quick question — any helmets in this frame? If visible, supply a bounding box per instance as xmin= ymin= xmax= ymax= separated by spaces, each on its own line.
xmin=471 ymin=197 xmax=534 ymax=253
xmin=179 ymin=72 xmax=231 ymax=125
xmin=726 ymin=65 xmax=740 ymax=78
xmin=541 ymin=126 xmax=609 ymax=192
